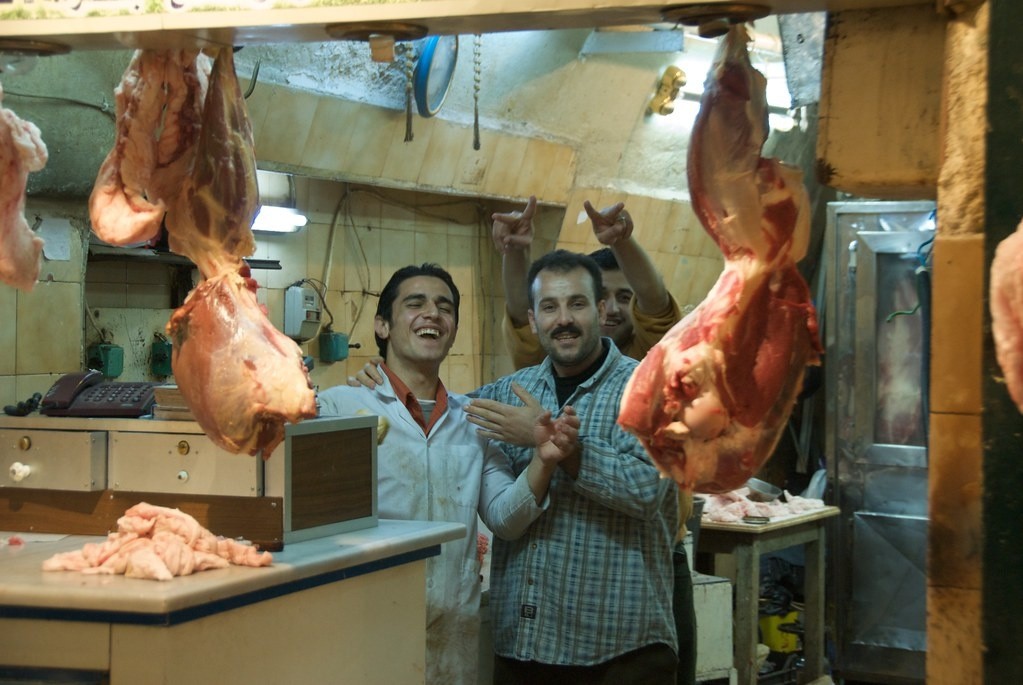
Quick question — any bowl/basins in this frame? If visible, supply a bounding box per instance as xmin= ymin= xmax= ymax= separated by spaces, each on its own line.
xmin=746 ymin=478 xmax=782 ymax=502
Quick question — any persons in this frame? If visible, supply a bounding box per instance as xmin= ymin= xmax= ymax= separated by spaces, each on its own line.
xmin=491 ymin=195 xmax=698 ymax=685
xmin=344 ymin=249 xmax=678 ymax=685
xmin=317 ymin=262 xmax=581 ymax=685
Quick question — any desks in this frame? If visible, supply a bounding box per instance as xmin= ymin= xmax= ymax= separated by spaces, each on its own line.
xmin=697 ymin=504 xmax=842 ymax=685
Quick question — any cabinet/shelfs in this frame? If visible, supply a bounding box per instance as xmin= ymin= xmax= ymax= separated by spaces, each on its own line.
xmin=0 ymin=408 xmax=378 ymax=546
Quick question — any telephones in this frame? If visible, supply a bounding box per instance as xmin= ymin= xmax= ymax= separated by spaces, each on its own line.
xmin=38 ymin=370 xmax=162 ymax=419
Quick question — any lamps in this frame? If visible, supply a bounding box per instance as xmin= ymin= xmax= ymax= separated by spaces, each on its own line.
xmin=252 ymin=206 xmax=307 ymax=234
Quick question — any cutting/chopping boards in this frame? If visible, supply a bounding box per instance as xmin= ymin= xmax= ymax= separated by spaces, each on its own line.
xmin=700 ymin=507 xmax=834 ymax=528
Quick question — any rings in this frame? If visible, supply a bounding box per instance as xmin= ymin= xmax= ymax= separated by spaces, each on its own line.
xmin=616 ymin=217 xmax=625 ymax=220
